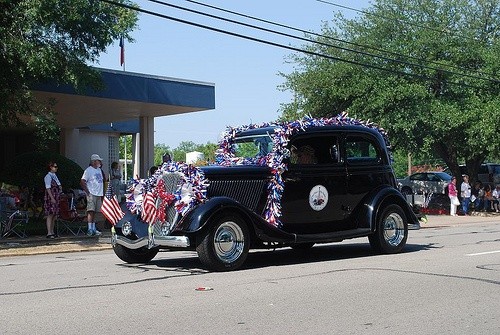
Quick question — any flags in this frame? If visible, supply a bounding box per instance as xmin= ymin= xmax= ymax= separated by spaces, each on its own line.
xmin=119 ymin=34 xmax=125 ymax=67
xmin=100 ymin=181 xmax=125 ymax=226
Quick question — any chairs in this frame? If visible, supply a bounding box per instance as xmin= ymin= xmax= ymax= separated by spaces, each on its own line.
xmin=55 ymin=187 xmax=88 ymax=237
xmin=0 ymin=183 xmax=30 ymax=240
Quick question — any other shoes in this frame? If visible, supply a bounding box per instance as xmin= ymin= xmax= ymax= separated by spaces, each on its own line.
xmin=52 ymin=233 xmax=58 ymax=237
xmin=93 ymin=229 xmax=102 ymax=236
xmin=47 ymin=234 xmax=55 ymax=239
xmin=87 ymin=230 xmax=99 ymax=238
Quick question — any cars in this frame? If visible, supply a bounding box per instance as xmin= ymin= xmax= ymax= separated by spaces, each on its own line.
xmin=112 ymin=119 xmax=421 ymax=272
xmin=396 ymin=163 xmax=500 ymax=197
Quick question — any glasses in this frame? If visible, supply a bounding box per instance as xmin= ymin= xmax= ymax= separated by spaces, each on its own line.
xmin=297 ymin=154 xmax=306 ymax=157
xmin=51 ymin=165 xmax=58 ymax=168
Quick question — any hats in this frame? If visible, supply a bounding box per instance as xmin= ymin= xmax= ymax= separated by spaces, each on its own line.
xmin=91 ymin=154 xmax=103 ymax=161
xmin=292 ymin=145 xmax=314 ymax=156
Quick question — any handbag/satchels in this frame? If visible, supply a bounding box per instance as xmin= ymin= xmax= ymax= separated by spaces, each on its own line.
xmin=452 ymin=196 xmax=461 ymax=206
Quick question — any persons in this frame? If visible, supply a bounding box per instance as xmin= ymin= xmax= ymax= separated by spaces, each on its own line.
xmin=461 ymin=174 xmax=471 ymax=216
xmin=111 ymin=162 xmax=122 ymax=180
xmin=448 ymin=176 xmax=459 ymax=216
xmin=43 ymin=160 xmax=64 ymax=239
xmin=470 ymin=179 xmax=500 ymax=213
xmin=80 ymin=154 xmax=104 ymax=237
xmin=98 ymin=160 xmax=105 ymax=182
xmin=292 ymin=146 xmax=316 ymax=165
